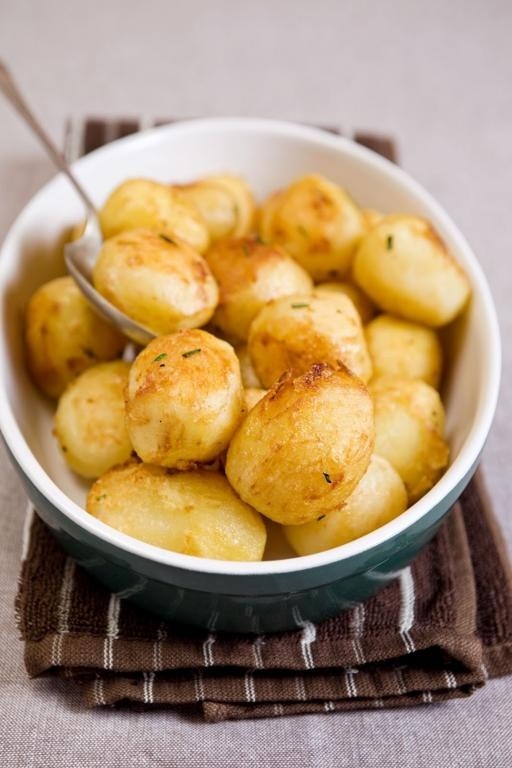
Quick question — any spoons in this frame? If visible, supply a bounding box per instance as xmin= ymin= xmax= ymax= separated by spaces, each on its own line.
xmin=0 ymin=66 xmax=154 ymax=344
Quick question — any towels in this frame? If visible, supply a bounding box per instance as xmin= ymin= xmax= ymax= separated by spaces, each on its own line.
xmin=17 ymin=97 xmax=512 ymax=726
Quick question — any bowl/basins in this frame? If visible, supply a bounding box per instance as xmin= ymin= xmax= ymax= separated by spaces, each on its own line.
xmin=0 ymin=116 xmax=505 ymax=635
